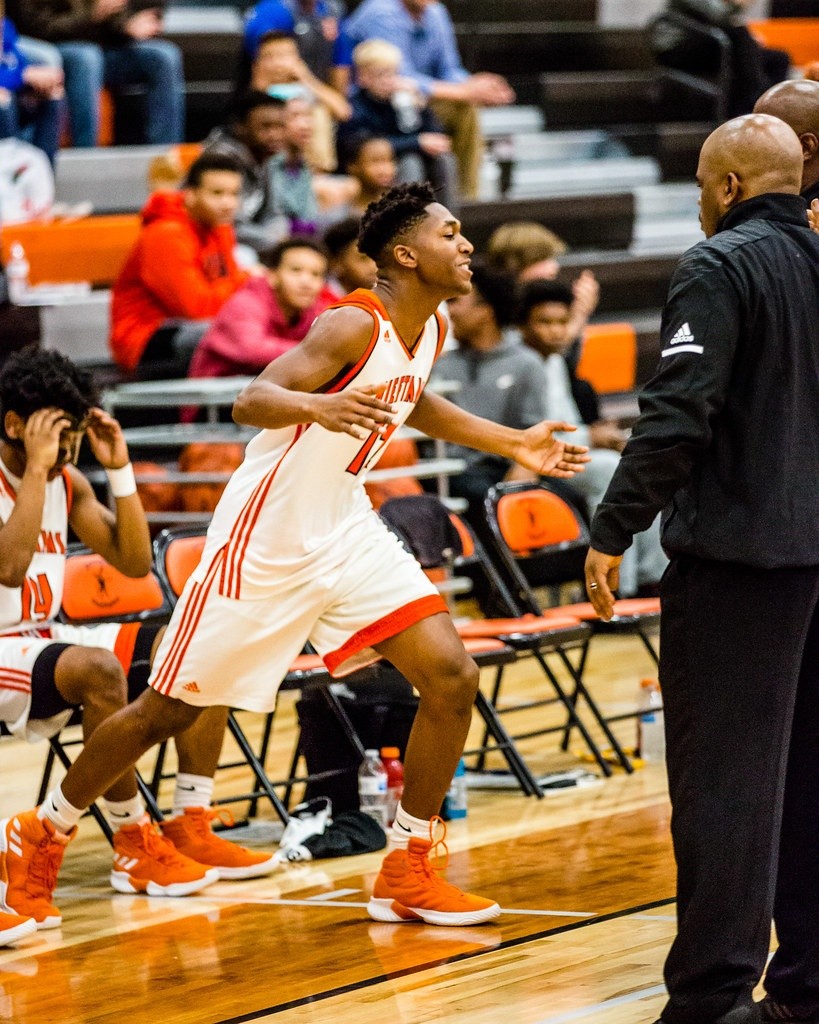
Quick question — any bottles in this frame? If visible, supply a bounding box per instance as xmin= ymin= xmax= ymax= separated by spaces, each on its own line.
xmin=634 ymin=679 xmax=665 ymax=762
xmin=391 ymin=90 xmax=422 ymax=133
xmin=357 ymin=749 xmax=388 ymax=832
xmin=445 ymin=758 xmax=468 ymax=819
xmin=7 ymin=244 xmax=30 ymax=304
xmin=381 ymin=747 xmax=403 ymax=828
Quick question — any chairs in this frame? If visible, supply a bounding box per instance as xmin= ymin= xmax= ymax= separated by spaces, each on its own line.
xmin=2 ymin=477 xmax=660 ymax=882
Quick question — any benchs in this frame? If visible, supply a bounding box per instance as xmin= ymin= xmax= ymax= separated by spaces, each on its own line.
xmin=1 ymin=1 xmax=819 ymax=589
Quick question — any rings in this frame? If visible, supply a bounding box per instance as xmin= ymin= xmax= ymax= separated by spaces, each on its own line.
xmin=590 ymin=582 xmax=598 ymax=590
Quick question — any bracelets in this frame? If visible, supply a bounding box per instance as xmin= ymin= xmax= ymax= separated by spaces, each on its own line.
xmin=105 ymin=462 xmax=137 ymax=498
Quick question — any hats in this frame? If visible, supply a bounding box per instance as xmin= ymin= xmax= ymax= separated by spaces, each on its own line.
xmin=515 ymin=278 xmax=573 ymax=322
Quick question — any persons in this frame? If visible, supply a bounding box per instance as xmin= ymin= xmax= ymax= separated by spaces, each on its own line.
xmin=0 ymin=179 xmax=595 ymax=931
xmin=0 ymin=344 xmax=284 ymax=899
xmin=581 ymin=79 xmax=819 ymax=1024
xmin=0 ymin=1 xmax=669 ymax=633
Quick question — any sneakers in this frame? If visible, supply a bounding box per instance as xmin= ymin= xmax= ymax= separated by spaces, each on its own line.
xmin=0 ymin=912 xmax=37 ymax=949
xmin=109 ymin=812 xmax=221 ymax=897
xmin=368 ymin=922 xmax=502 ymax=970
xmin=112 ymin=893 xmax=158 ymax=952
xmin=0 ymin=808 xmax=79 ymax=930
xmin=157 ymin=807 xmax=280 ymax=880
xmin=364 ymin=815 xmax=502 ymax=927
xmin=145 ymin=882 xmax=283 ymax=939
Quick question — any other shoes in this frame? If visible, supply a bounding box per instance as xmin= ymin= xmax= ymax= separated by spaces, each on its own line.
xmin=588 ymin=618 xmax=640 ymax=634
xmin=757 ymin=992 xmax=800 ymax=1024
